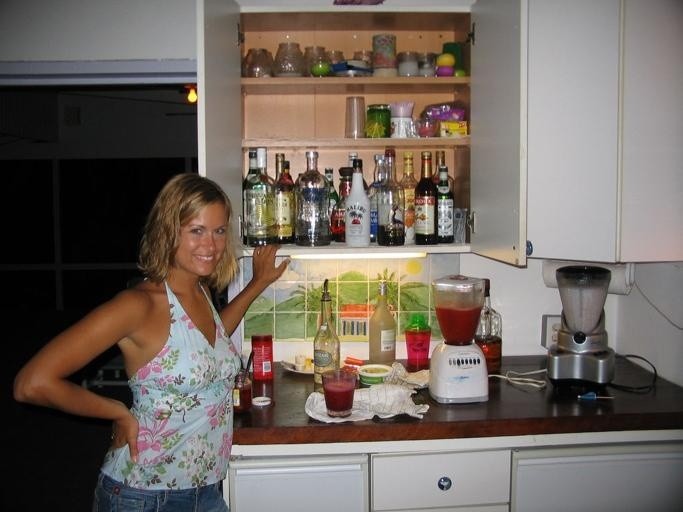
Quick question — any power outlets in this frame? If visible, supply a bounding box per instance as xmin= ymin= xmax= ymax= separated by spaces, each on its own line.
xmin=543 ymin=315 xmax=564 ymax=350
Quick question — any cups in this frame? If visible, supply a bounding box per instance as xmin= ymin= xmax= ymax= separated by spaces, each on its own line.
xmin=413 ymin=119 xmax=440 ymax=139
xmin=452 ymin=207 xmax=467 ymax=244
xmin=345 ymin=98 xmax=366 ymax=138
xmin=320 ymin=371 xmax=356 ymax=417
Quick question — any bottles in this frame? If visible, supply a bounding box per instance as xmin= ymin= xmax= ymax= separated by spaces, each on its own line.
xmin=475 ymin=280 xmax=501 ymax=373
xmin=242 ymin=146 xmax=455 ymax=247
xmin=369 ymin=279 xmax=397 ymax=367
xmin=312 ymin=282 xmax=340 ymax=383
xmin=401 ymin=313 xmax=430 ymax=367
xmin=250 ymin=333 xmax=273 ymax=382
xmin=242 ymin=35 xmax=436 ymax=79
xmin=363 ymin=103 xmax=390 ymax=138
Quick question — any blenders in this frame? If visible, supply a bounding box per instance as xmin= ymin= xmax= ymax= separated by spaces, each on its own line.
xmin=429 ymin=275 xmax=489 ymax=403
xmin=547 ymin=266 xmax=616 ymax=387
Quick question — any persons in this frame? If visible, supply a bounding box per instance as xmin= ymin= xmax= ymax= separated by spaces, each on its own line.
xmin=13 ymin=172 xmax=290 ymax=512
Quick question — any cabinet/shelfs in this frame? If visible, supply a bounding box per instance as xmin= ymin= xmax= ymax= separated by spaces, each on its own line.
xmin=219 ymin=442 xmax=370 ymax=511
xmin=510 ymin=430 xmax=682 ymax=511
xmin=370 ymin=439 xmax=510 ymax=511
xmin=525 ymin=1 xmax=682 ymax=262
xmin=194 ymin=0 xmax=527 ymax=268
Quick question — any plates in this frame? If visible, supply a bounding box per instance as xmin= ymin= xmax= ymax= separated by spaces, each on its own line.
xmin=282 ymin=362 xmax=344 ymax=375
